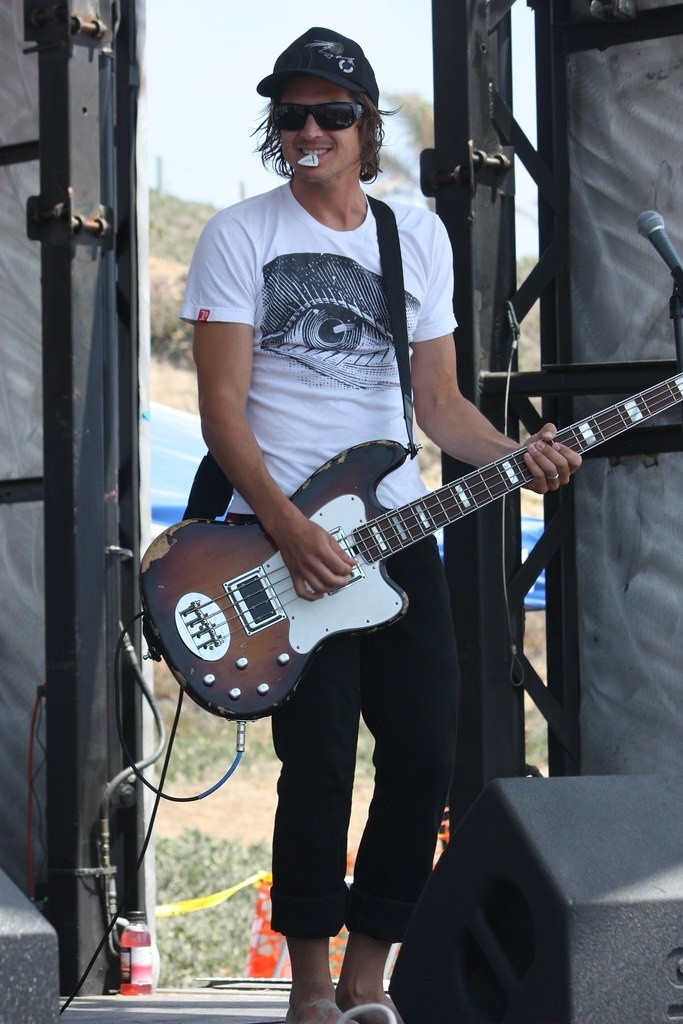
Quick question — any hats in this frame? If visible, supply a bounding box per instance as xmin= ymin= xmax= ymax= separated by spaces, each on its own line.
xmin=256 ymin=27 xmax=379 ymax=110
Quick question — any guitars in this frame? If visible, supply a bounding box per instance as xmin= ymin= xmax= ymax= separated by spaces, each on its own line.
xmin=138 ymin=372 xmax=683 ymax=723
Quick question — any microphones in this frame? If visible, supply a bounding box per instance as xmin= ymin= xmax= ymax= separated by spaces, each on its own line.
xmin=637 ymin=210 xmax=683 ymax=287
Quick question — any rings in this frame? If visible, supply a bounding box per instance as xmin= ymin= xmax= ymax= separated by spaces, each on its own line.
xmin=546 ymin=474 xmax=560 ymax=481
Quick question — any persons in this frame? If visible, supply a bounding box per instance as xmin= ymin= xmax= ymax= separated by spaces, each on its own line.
xmin=174 ymin=28 xmax=582 ymax=1024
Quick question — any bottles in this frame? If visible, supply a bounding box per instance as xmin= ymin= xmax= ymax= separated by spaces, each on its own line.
xmin=118 ymin=911 xmax=153 ymax=996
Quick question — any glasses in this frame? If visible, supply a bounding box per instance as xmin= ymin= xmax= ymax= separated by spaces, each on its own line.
xmin=270 ymin=102 xmax=365 ymax=131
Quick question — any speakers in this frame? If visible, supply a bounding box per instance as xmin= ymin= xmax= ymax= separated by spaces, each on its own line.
xmin=0 ymin=868 xmax=61 ymax=1024
xmin=389 ymin=775 xmax=683 ymax=1024
